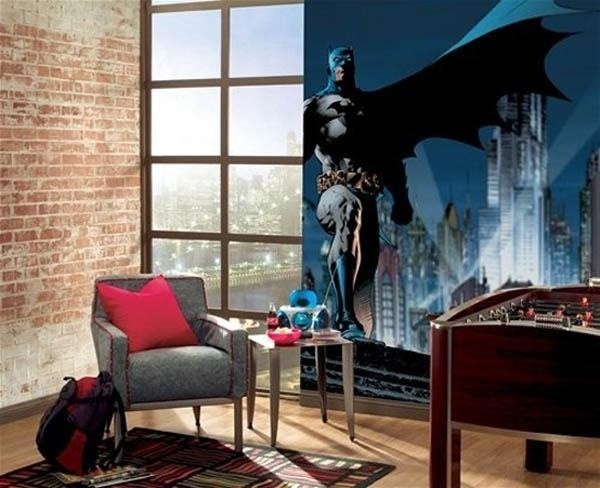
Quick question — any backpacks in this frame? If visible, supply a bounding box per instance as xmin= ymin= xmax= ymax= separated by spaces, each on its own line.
xmin=34 ymin=369 xmax=128 ymax=476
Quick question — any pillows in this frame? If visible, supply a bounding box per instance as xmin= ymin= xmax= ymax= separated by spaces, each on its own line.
xmin=95 ymin=275 xmax=199 ymax=352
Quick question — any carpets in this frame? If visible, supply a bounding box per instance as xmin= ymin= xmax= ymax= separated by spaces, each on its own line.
xmin=0 ymin=426 xmax=397 ymax=488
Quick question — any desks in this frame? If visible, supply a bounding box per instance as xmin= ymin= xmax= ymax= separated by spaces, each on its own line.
xmin=246 ymin=328 xmax=355 ymax=447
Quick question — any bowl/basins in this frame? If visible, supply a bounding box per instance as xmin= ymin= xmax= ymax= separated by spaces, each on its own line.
xmin=266 ymin=329 xmax=303 ymax=346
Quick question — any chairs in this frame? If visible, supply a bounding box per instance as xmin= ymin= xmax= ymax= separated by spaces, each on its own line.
xmin=90 ymin=273 xmax=250 ymax=465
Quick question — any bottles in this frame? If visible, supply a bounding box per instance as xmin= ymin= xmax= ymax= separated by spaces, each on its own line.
xmin=267 ymin=302 xmax=279 ymax=330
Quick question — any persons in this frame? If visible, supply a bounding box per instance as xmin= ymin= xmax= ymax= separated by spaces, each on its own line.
xmin=302 ymin=41 xmax=416 ymax=342
